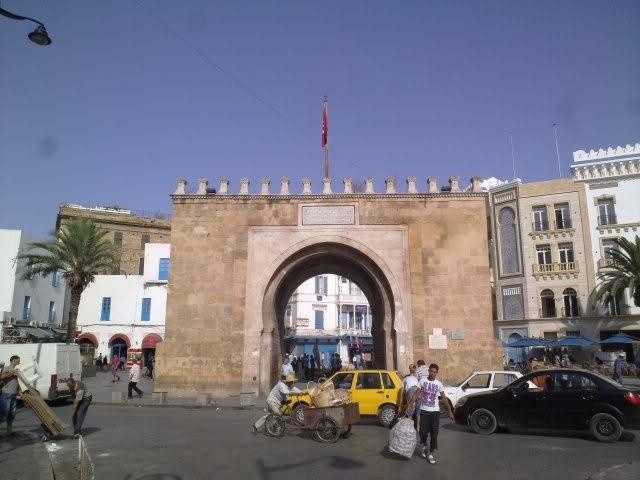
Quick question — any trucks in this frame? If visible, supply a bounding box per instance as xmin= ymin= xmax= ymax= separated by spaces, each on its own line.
xmin=0 ymin=343 xmax=83 ymax=408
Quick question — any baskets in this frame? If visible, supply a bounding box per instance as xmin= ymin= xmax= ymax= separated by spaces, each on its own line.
xmin=307 ymin=377 xmax=352 ymax=408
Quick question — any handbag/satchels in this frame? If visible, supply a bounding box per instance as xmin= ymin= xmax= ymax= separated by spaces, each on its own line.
xmin=389 ymin=418 xmax=417 ymax=459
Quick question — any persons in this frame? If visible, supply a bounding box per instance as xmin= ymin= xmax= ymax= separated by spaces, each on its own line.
xmin=66 ymin=377 xmax=93 ymax=438
xmin=280 ymin=352 xmax=429 ymax=433
xmin=404 ymin=363 xmax=456 ymax=466
xmin=504 ymin=332 xmax=640 ymax=392
xmin=0 ymin=362 xmax=6 ymax=371
xmin=251 ymin=373 xmax=307 ymax=435
xmin=0 ymin=356 xmax=24 ymax=438
xmin=97 ymin=352 xmax=156 ymax=400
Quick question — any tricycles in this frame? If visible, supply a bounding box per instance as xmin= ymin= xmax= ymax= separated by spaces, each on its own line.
xmin=264 ymin=392 xmax=361 ymax=443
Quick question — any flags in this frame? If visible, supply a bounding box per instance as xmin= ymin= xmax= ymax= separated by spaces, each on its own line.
xmin=320 ymin=101 xmax=329 ymax=150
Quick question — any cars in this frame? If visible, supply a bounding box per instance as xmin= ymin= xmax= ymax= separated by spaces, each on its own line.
xmin=453 ymin=366 xmax=640 ymax=443
xmin=280 ymin=369 xmax=407 ymax=429
xmin=437 ymin=369 xmax=538 ymax=418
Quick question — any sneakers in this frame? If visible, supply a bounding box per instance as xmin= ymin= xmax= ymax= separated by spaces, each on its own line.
xmin=420 ymin=446 xmax=436 ymax=464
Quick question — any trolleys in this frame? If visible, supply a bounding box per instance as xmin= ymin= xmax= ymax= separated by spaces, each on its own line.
xmin=13 ymin=368 xmax=67 ymax=442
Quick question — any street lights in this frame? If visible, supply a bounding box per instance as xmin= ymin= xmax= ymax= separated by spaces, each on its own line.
xmin=0 ymin=8 xmax=53 ymax=46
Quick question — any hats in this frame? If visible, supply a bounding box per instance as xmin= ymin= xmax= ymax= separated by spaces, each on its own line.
xmin=282 ymin=375 xmax=299 ymax=382
